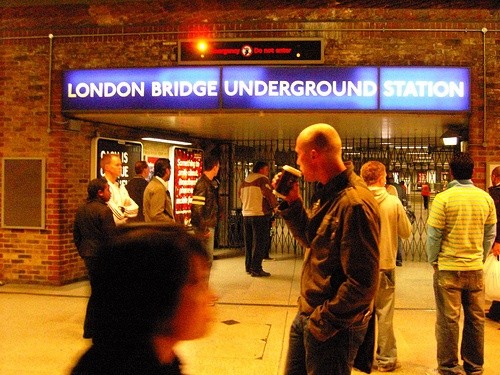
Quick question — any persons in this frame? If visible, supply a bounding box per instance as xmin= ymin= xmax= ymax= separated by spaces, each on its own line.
xmin=272 ymin=124 xmax=381 ymax=375
xmin=143 ymin=158 xmax=176 ymax=224
xmin=424 ymin=152 xmax=498 ymax=375
xmin=421 ymin=179 xmax=430 ymax=210
xmin=189 ymin=156 xmax=220 ymax=265
xmin=384 ymin=182 xmax=399 ymax=198
xmin=100 ymin=153 xmax=139 ymax=227
xmin=386 ymin=172 xmax=407 ymax=266
xmin=484 ymin=164 xmax=500 ymax=323
xmin=72 ymin=177 xmax=117 ymax=339
xmin=69 ymin=220 xmax=217 ymax=375
xmin=400 ymin=181 xmax=406 ymax=191
xmin=358 ymin=160 xmax=412 ymax=372
xmin=237 ymin=161 xmax=280 ymax=277
xmin=125 ymin=161 xmax=150 ymax=222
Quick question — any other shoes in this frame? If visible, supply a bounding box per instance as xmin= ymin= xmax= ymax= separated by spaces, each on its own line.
xmin=426 ymin=365 xmax=441 ymax=375
xmin=249 ymin=269 xmax=270 ymax=277
xmin=379 ymin=361 xmax=402 ymax=372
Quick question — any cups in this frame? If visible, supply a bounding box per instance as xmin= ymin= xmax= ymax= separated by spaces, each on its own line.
xmin=272 ymin=165 xmax=302 ymax=199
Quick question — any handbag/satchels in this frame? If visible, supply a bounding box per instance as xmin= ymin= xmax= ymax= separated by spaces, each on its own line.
xmin=482 ymin=254 xmax=500 ymax=300
